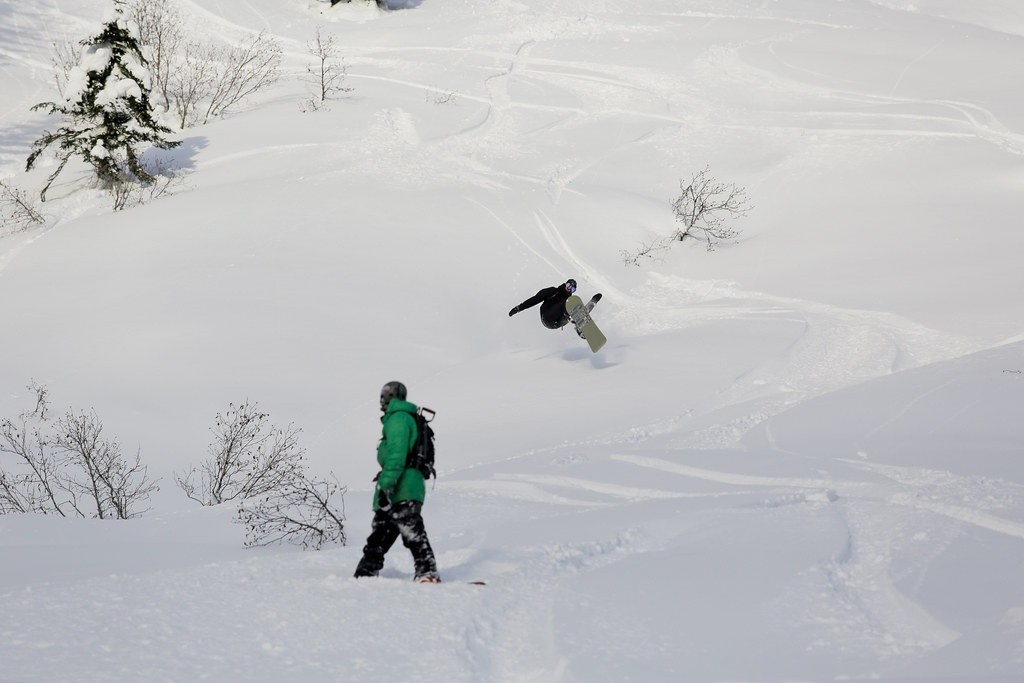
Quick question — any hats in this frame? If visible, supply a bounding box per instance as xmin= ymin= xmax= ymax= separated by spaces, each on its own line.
xmin=566 ymin=279 xmax=577 ymax=288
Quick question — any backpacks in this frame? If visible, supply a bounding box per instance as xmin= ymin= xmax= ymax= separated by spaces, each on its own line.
xmin=391 ymin=407 xmax=436 ymax=480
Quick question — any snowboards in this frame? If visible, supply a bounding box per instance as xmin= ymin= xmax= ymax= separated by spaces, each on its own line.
xmin=565 ymin=295 xmax=607 ymax=353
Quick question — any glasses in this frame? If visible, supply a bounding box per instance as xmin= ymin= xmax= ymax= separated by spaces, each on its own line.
xmin=567 ymin=283 xmax=576 ymax=292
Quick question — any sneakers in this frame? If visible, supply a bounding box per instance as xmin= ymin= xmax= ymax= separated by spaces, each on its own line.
xmin=414 ymin=567 xmax=440 ymax=582
xmin=356 ymin=557 xmax=383 ymax=577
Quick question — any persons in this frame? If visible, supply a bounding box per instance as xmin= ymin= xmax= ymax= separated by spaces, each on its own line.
xmin=354 ymin=382 xmax=441 ymax=583
xmin=509 ymin=279 xmax=603 ymax=339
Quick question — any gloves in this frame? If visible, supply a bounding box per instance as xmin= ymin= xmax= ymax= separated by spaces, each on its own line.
xmin=509 ymin=306 xmax=519 ymax=316
xmin=378 ymin=490 xmax=392 ymax=510
